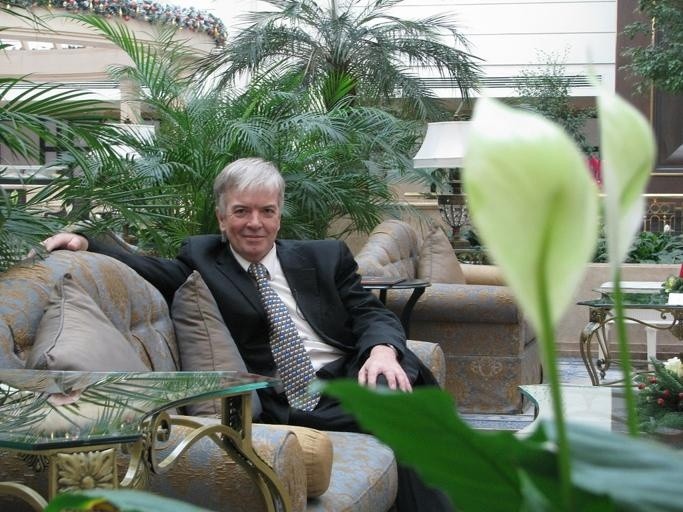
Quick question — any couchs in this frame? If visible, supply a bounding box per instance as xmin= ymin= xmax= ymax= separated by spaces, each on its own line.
xmin=0 ymin=249 xmax=447 ymax=512
xmin=353 ymin=219 xmax=546 ymax=415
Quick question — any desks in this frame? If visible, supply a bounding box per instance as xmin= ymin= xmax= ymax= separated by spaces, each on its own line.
xmin=599 ymin=281 xmax=683 ymax=371
xmin=359 ymin=279 xmax=432 ymax=340
xmin=598 ymin=282 xmax=669 ymax=370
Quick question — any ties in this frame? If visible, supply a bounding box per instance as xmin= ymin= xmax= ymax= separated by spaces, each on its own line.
xmin=248 ymin=261 xmax=323 ymax=412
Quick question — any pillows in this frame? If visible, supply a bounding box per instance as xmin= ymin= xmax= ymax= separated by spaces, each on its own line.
xmin=172 ymin=271 xmax=263 ymax=420
xmin=25 ymin=274 xmax=148 ymax=371
xmin=278 ymin=425 xmax=334 ymax=499
xmin=418 ymin=227 xmax=465 ymax=285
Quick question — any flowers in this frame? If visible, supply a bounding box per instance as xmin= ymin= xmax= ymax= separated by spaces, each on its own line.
xmin=632 ymin=351 xmax=683 ymax=435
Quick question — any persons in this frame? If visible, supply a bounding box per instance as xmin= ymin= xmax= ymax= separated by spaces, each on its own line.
xmin=28 ymin=157 xmax=450 ymax=512
